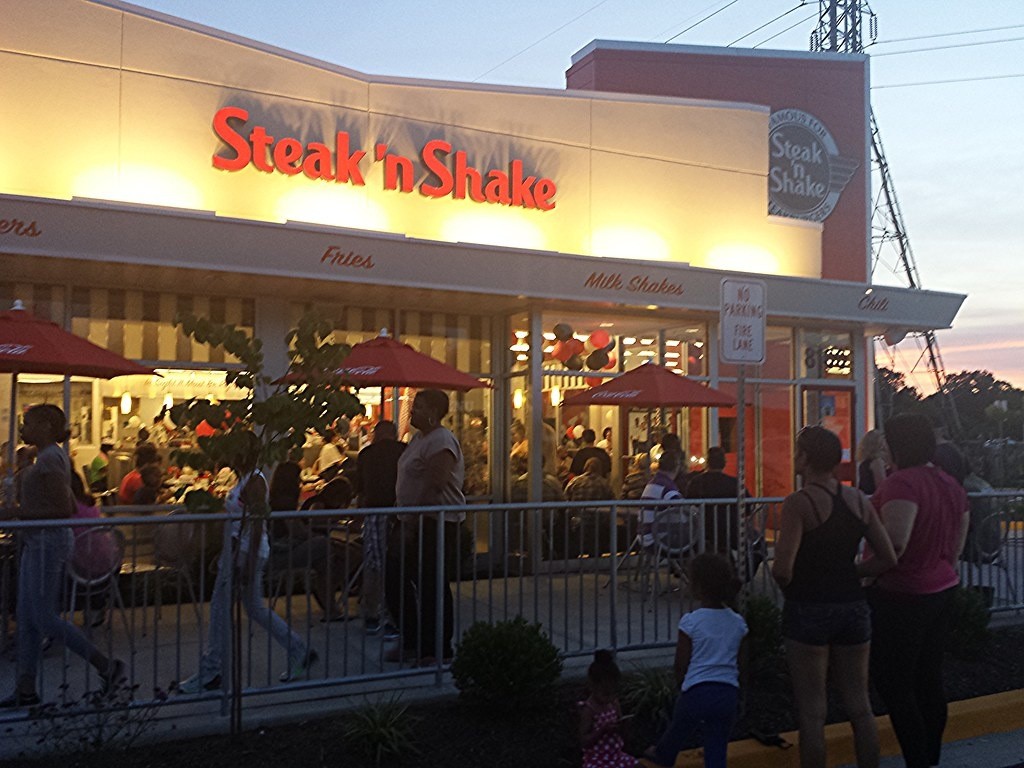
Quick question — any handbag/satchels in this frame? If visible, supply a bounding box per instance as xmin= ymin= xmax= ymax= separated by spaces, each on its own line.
xmin=67 ymin=489 xmax=118 ymax=577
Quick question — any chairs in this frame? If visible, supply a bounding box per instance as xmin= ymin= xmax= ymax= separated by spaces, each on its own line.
xmin=0 ymin=445 xmax=1021 ymax=632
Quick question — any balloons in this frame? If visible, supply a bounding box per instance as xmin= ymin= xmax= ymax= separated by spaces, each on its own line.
xmin=550 ymin=323 xmax=616 ymax=387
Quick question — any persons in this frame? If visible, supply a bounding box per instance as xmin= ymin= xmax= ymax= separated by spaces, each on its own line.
xmin=862 ymin=411 xmax=970 ymax=768
xmin=0 ymin=389 xmax=768 ymax=708
xmin=856 ymin=429 xmax=888 ymax=495
xmin=579 ymin=649 xmax=640 ymax=768
xmin=643 ymin=554 xmax=750 ymax=768
xmin=772 ymin=426 xmax=898 ymax=768
xmin=929 ymin=416 xmax=968 ymax=485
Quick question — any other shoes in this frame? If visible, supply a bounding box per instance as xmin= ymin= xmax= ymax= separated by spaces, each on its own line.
xmin=412 ymin=647 xmax=452 ymax=669
xmin=381 ymin=644 xmax=419 ymax=662
xmin=0 ymin=688 xmax=41 ymax=710
xmin=279 ymin=657 xmax=322 ymax=681
xmin=179 ymin=670 xmax=224 ymax=693
xmin=95 ymin=658 xmax=128 ymax=701
xmin=366 ymin=612 xmax=390 ymax=632
xmin=644 ymin=746 xmax=659 ymax=764
xmin=384 ymin=623 xmax=402 ymax=638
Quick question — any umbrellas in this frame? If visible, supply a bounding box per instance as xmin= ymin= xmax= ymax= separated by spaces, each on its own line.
xmin=558 ymin=360 xmax=753 ymax=452
xmin=0 ymin=300 xmax=164 ymax=509
xmin=270 ymin=326 xmax=500 ymax=421
